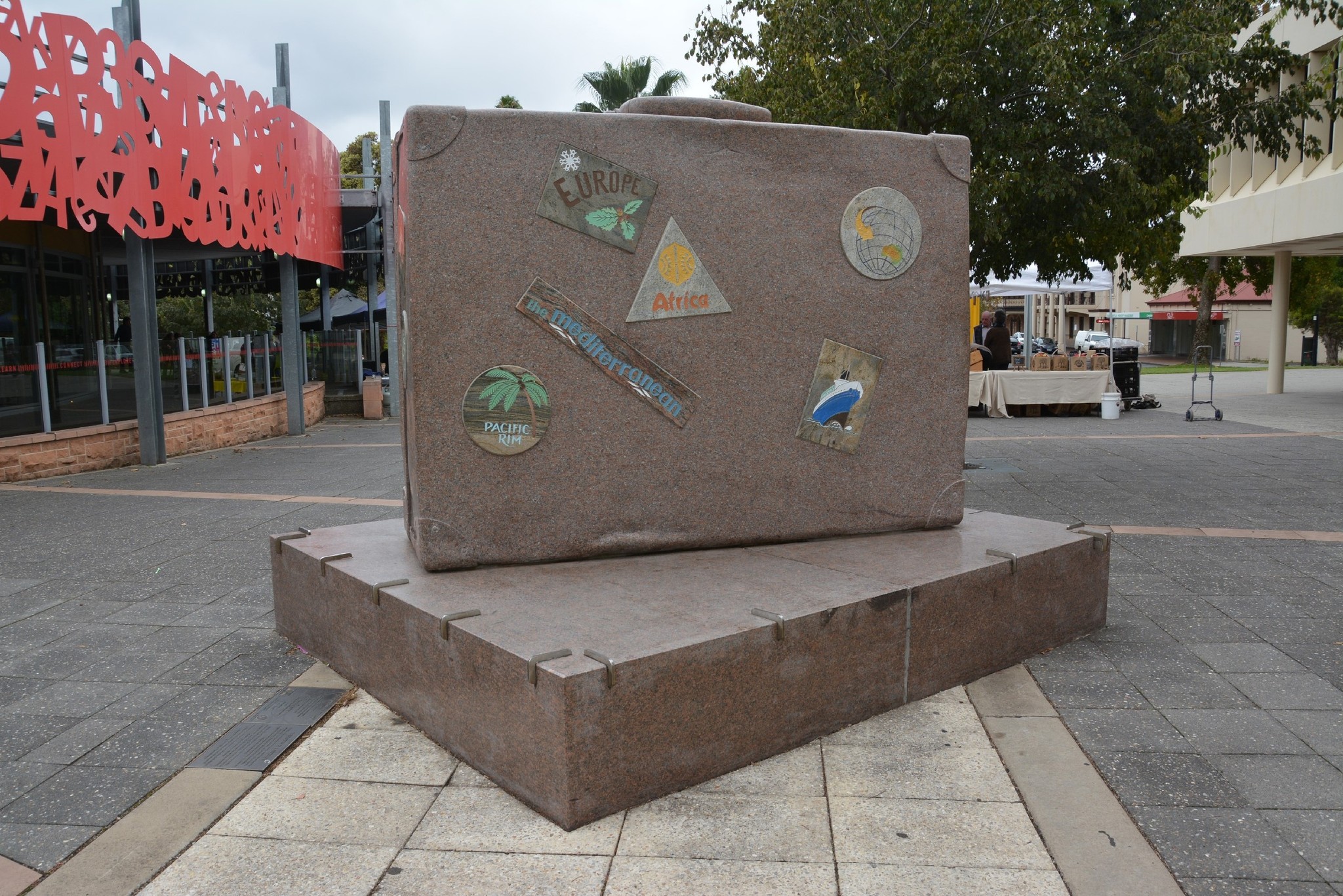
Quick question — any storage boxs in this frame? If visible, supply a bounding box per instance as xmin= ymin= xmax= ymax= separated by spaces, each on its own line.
xmin=970 ymin=348 xmax=1140 ymax=417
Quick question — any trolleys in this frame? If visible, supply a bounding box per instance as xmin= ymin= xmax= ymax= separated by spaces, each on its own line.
xmin=1185 ymin=345 xmax=1223 ymax=422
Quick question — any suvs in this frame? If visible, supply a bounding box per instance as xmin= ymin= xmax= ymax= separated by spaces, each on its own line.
xmin=1013 ymin=332 xmax=1036 ymax=350
xmin=1010 ymin=336 xmax=1022 ymax=354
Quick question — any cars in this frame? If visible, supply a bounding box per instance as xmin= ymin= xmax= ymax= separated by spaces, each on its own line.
xmin=54 ymin=344 xmax=133 ymax=367
xmin=1032 ymin=337 xmax=1058 ymax=355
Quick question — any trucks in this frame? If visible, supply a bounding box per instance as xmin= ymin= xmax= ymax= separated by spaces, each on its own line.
xmin=1074 ymin=330 xmax=1110 ymax=354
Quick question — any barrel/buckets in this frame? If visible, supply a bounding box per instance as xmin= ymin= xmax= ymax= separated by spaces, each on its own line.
xmin=1101 ymin=383 xmax=1121 ymax=419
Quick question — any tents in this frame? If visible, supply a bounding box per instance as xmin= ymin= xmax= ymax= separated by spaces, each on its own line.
xmin=299 ymin=288 xmax=386 ymax=332
xmin=970 ymin=244 xmax=1114 ymax=418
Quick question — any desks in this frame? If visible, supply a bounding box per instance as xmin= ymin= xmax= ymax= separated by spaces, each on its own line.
xmin=252 ymin=355 xmax=274 ymax=382
xmin=214 ymin=377 xmax=280 ymax=398
xmin=968 ymin=370 xmax=1118 ymax=419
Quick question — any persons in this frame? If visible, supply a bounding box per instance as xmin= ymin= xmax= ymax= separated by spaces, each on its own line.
xmin=973 ymin=311 xmax=994 ymax=411
xmin=209 ymin=332 xmax=222 ymax=339
xmin=984 ymin=310 xmax=1012 ymax=371
xmin=115 ymin=316 xmax=133 ymax=373
xmin=162 ymin=332 xmax=193 ymax=378
xmin=240 ymin=335 xmax=282 ymax=380
xmin=5 ymin=341 xmax=18 ymax=366
